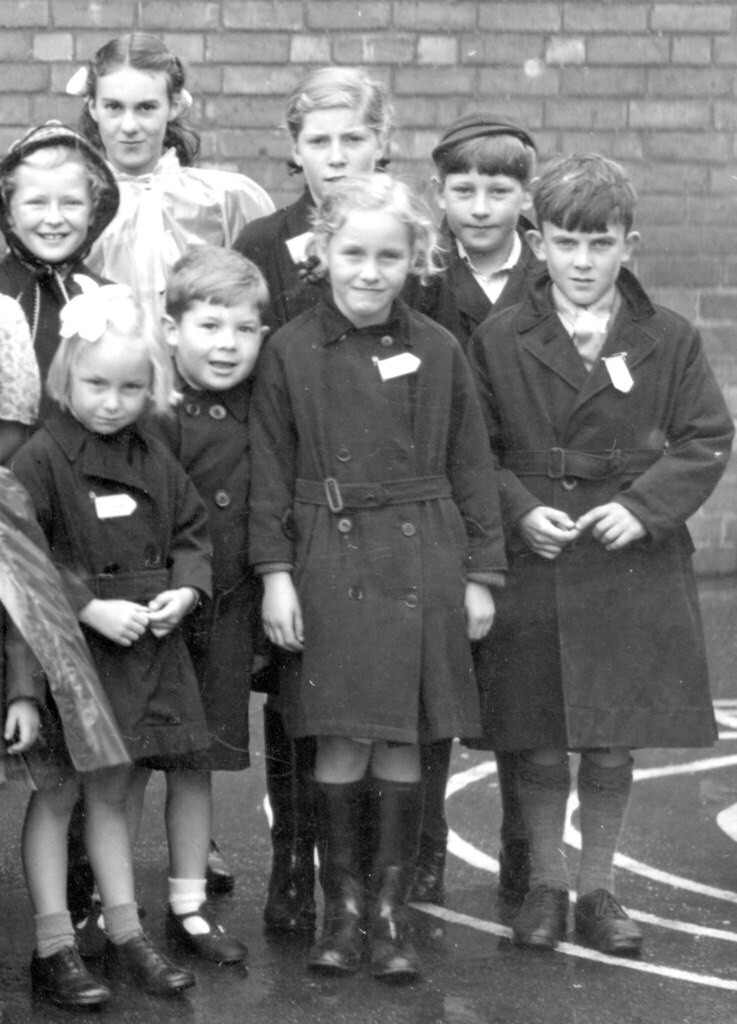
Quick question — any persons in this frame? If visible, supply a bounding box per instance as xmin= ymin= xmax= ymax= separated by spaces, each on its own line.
xmin=77 ymin=31 xmax=279 ymax=892
xmin=248 ymin=173 xmax=508 ymax=983
xmin=0 ymin=119 xmax=150 ymax=943
xmin=0 ymin=249 xmax=270 ymax=1012
xmin=235 ymin=67 xmax=418 ymax=935
xmin=466 ymin=153 xmax=734 ymax=956
xmin=408 ymin=114 xmax=552 ymax=904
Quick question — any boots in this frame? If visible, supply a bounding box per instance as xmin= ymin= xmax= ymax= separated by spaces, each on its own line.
xmin=263 ymin=703 xmax=317 ymax=933
xmin=308 ymin=777 xmax=427 ymax=976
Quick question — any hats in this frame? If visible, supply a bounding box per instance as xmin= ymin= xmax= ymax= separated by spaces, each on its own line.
xmin=433 ymin=110 xmax=538 ymax=162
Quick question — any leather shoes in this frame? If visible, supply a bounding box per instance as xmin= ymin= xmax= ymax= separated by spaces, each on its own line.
xmin=411 ymin=841 xmax=447 ymax=902
xmin=104 ymin=930 xmax=196 ymax=994
xmin=166 ymin=900 xmax=250 ymax=961
xmin=500 ymin=839 xmax=530 ymax=893
xmin=573 ymin=889 xmax=644 ymax=951
xmin=512 ymin=885 xmax=569 ymax=948
xmin=29 ymin=944 xmax=112 ymax=1005
xmin=75 ymin=899 xmax=108 ymax=955
xmin=207 ymin=839 xmax=234 ymax=893
